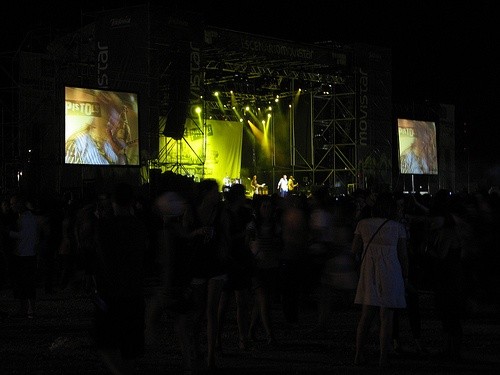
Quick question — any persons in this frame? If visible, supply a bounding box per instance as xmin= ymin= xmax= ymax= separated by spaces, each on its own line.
xmin=400 ymin=121 xmax=438 ymax=174
xmin=65 ymin=92 xmax=131 ymax=165
xmin=0 ymin=151 xmax=500 ymax=375
